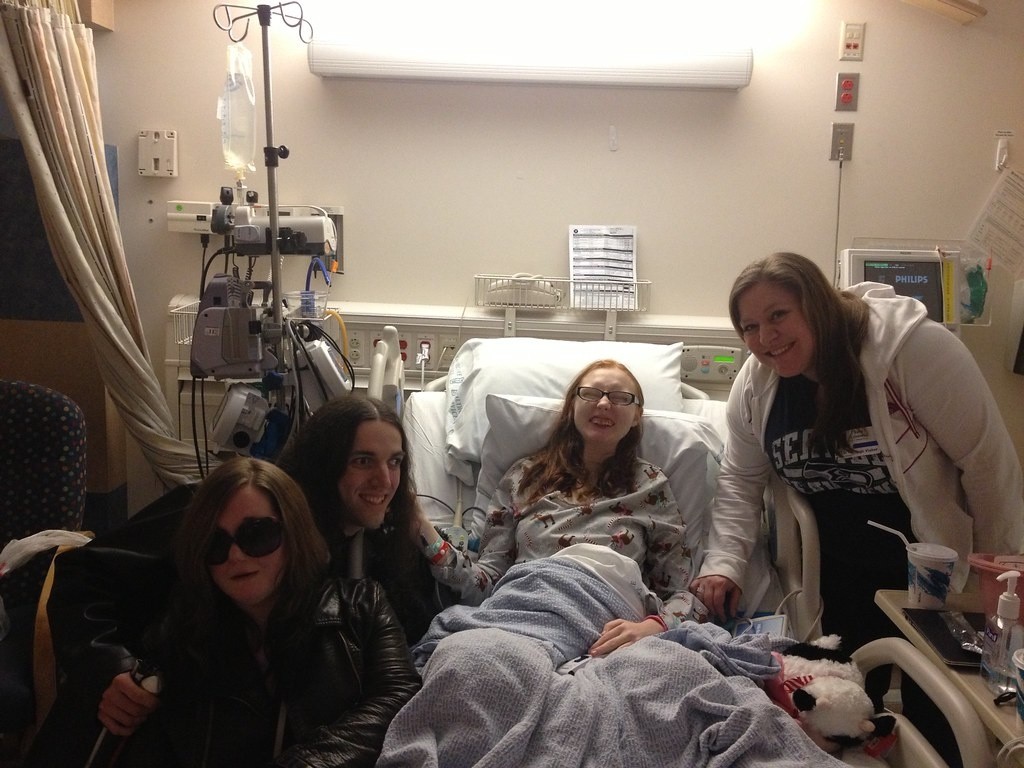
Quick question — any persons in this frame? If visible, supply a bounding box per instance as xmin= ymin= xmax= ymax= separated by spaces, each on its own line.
xmin=277 ymin=396 xmax=444 ymax=651
xmin=70 ymin=457 xmax=423 ymax=768
xmin=406 ymin=360 xmax=708 ymax=658
xmin=690 ymin=252 xmax=1024 ymax=768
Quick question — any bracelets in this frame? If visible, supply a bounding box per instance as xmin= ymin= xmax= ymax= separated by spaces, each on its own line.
xmin=424 ymin=536 xmax=455 ymax=567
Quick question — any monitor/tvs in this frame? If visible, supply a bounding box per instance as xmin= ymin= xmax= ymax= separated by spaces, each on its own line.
xmin=840 ymin=249 xmax=961 ymax=338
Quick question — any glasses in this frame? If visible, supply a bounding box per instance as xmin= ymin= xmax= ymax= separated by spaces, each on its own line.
xmin=202 ymin=515 xmax=283 ymax=566
xmin=574 ymin=386 xmax=642 ymax=406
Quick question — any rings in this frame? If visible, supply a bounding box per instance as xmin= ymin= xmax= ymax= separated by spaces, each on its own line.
xmin=696 ymin=587 xmax=705 ymax=594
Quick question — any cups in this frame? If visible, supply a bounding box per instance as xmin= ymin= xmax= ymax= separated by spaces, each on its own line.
xmin=1012 ymin=649 xmax=1024 ymax=727
xmin=906 ymin=542 xmax=959 ymax=612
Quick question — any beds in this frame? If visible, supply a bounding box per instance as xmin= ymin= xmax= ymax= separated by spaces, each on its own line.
xmin=401 ymin=337 xmax=992 ymax=768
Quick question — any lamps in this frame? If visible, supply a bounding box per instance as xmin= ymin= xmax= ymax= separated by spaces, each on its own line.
xmin=308 ymin=34 xmax=754 ymax=93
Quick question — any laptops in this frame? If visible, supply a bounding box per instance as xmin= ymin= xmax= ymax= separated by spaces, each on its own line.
xmin=901 ymin=607 xmax=986 ymax=667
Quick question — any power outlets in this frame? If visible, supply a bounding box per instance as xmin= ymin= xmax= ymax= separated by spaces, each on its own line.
xmin=416 ymin=333 xmax=434 ymax=370
xmin=835 ymin=72 xmax=860 ymax=110
xmin=398 ymin=332 xmax=411 ymax=369
xmin=840 ymin=21 xmax=866 ymax=61
xmin=829 ymin=123 xmax=854 ymax=162
xmin=346 ymin=330 xmax=364 ymax=366
xmin=370 ymin=330 xmax=383 ymax=367
xmin=438 ymin=334 xmax=458 ymax=370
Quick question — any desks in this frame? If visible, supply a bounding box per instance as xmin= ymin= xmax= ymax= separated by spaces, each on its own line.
xmin=874 ymin=590 xmax=1024 ymax=768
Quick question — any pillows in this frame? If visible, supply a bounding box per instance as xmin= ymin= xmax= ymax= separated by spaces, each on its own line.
xmin=446 ymin=337 xmax=685 ymax=464
xmin=471 ymin=393 xmax=725 ymax=577
